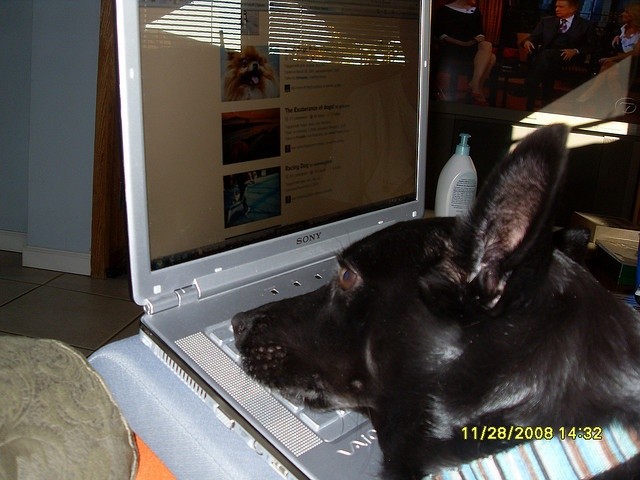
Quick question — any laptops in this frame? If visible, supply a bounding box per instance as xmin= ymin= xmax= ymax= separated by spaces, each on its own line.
xmin=114 ymin=0 xmax=433 ymax=480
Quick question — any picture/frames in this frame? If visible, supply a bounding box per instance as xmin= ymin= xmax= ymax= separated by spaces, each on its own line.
xmin=430 ymin=0 xmax=640 ymax=138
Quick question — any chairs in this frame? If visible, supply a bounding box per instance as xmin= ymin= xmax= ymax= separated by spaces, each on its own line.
xmin=432 ymin=38 xmax=501 ymax=107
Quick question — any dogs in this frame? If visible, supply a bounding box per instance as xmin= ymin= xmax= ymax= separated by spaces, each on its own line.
xmin=223 ymin=46 xmax=280 ymax=102
xmin=231 ymin=123 xmax=640 ymax=480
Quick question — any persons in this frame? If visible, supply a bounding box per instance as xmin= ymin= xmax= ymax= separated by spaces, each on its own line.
xmin=238 ymin=171 xmax=257 ymax=215
xmin=435 ymin=0 xmax=496 ymax=105
xmin=598 ymin=1 xmax=640 ymax=74
xmin=520 ymin=0 xmax=598 ymax=110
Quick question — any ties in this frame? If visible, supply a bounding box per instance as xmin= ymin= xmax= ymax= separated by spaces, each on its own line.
xmin=559 ymin=19 xmax=568 ymax=33
